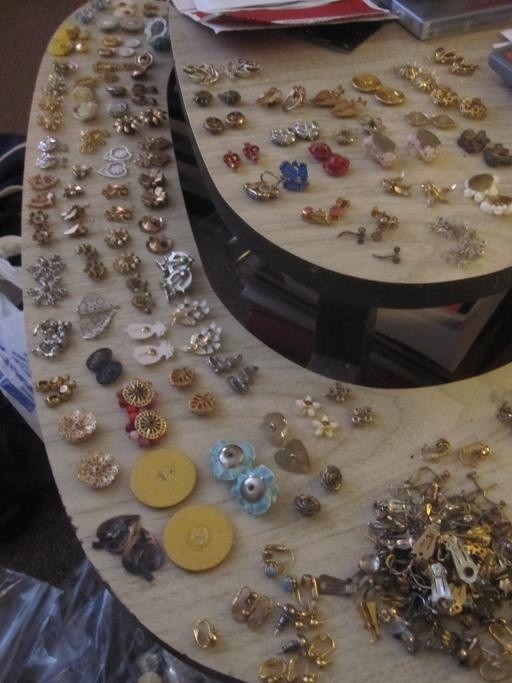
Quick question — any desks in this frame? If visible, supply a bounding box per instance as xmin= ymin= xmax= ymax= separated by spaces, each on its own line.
xmin=18 ymin=0 xmax=511 ymax=682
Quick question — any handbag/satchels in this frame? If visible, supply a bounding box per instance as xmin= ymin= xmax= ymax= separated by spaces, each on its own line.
xmin=0 ymin=234 xmax=44 ymax=444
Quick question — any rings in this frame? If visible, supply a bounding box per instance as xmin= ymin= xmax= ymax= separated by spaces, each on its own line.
xmin=193 ymin=536 xmax=336 ymax=682
xmin=480 ymin=654 xmax=512 ymax=682
xmin=416 ymin=437 xmax=490 ymax=462
xmin=281 ymin=85 xmax=306 ymax=112
xmin=183 ymin=55 xmax=262 ymax=86
xmin=433 ymin=46 xmax=478 ymax=75
xmin=485 ymin=614 xmax=512 ymax=650
